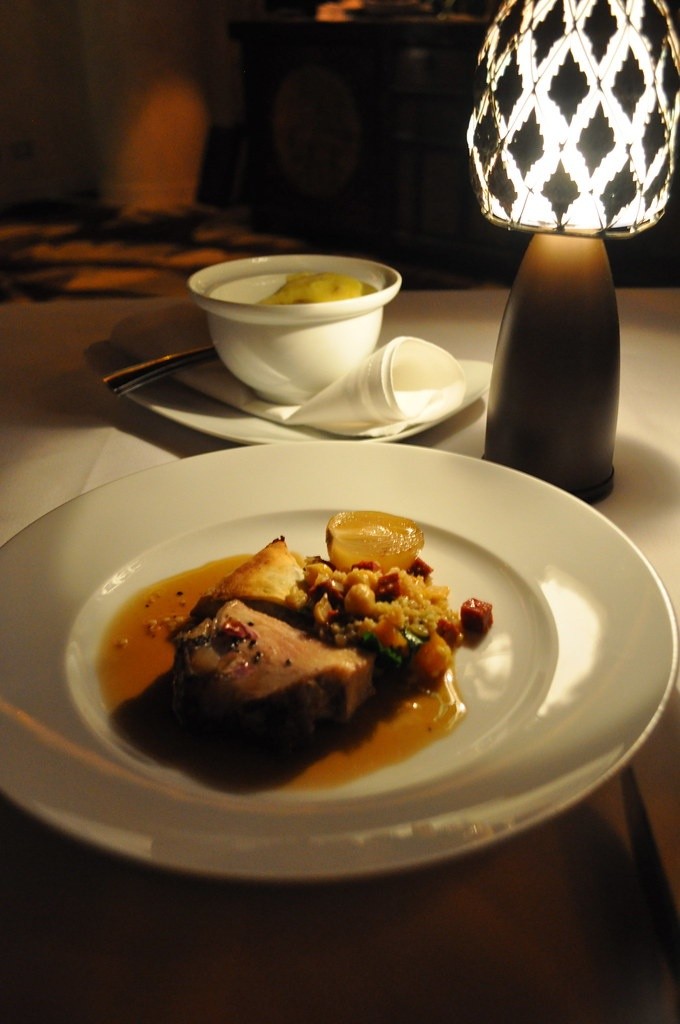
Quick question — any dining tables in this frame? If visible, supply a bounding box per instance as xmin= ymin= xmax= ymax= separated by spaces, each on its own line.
xmin=3 ymin=284 xmax=680 ymax=1022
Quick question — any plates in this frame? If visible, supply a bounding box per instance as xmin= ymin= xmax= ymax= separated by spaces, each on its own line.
xmin=87 ymin=342 xmax=495 ymax=446
xmin=0 ymin=437 xmax=678 ymax=885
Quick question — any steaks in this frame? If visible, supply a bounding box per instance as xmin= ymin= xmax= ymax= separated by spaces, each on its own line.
xmin=174 ymin=599 xmax=377 ymax=739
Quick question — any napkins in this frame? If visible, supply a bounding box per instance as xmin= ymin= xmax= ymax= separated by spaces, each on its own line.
xmin=108 ymin=302 xmax=468 ymax=440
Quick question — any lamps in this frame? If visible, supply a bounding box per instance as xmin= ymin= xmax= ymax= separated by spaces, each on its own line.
xmin=461 ymin=0 xmax=680 ymax=500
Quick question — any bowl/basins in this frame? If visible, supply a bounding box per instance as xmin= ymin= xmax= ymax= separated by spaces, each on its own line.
xmin=188 ymin=255 xmax=403 ymax=408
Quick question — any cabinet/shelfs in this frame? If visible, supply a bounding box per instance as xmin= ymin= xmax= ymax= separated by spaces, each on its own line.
xmin=225 ymin=4 xmax=528 ymax=280
